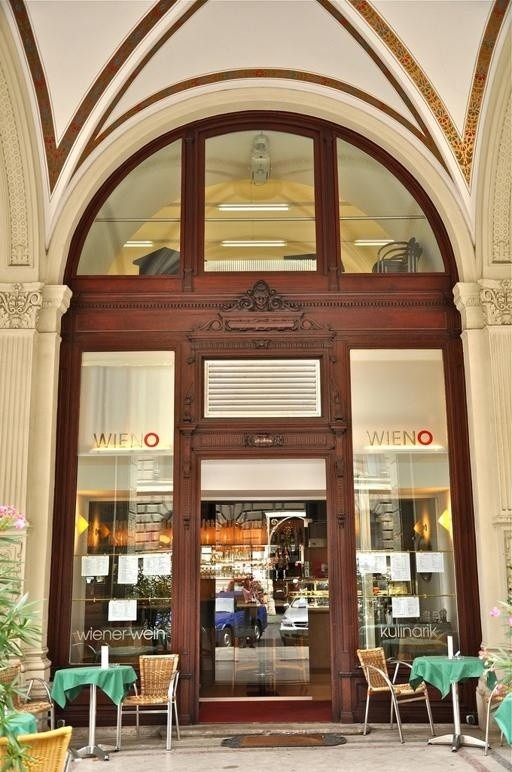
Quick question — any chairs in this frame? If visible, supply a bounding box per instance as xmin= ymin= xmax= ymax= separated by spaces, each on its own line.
xmin=116 ymin=654 xmax=180 ymax=750
xmin=0 ymin=726 xmax=73 ymax=772
xmin=0 ymin=665 xmax=56 ymax=730
xmin=372 ymin=237 xmax=423 ymax=273
xmin=484 ymin=685 xmax=512 ymax=756
xmin=357 ymin=647 xmax=435 ymax=744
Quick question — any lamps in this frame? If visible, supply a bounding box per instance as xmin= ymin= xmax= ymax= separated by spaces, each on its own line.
xmin=248 ymin=131 xmax=271 ymax=185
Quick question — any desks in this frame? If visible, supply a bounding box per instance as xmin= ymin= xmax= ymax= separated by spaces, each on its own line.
xmin=50 ymin=665 xmax=138 ymax=761
xmin=409 ymin=656 xmax=496 ymax=752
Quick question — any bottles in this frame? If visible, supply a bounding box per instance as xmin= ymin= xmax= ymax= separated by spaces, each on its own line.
xmin=201 ymin=564 xmax=246 ymax=576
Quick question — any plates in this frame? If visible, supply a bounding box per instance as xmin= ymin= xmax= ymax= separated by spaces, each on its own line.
xmin=452 ymin=656 xmax=464 ymax=660
xmin=108 ymin=662 xmax=121 ymax=668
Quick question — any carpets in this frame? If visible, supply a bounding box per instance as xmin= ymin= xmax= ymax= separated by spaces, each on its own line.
xmin=221 ymin=734 xmax=347 ymax=747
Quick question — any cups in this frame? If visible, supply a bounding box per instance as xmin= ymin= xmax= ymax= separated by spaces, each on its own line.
xmin=100 ymin=645 xmax=109 ymax=668
xmin=447 ymin=635 xmax=454 ymax=659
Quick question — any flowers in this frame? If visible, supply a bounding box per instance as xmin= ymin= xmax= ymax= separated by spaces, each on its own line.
xmin=0 ymin=504 xmax=46 ymax=772
xmin=479 ymin=596 xmax=512 ymax=709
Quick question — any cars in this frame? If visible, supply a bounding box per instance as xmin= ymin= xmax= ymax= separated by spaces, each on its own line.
xmin=280 ymin=579 xmax=332 ymax=646
xmin=212 ymin=593 xmax=267 ymax=647
